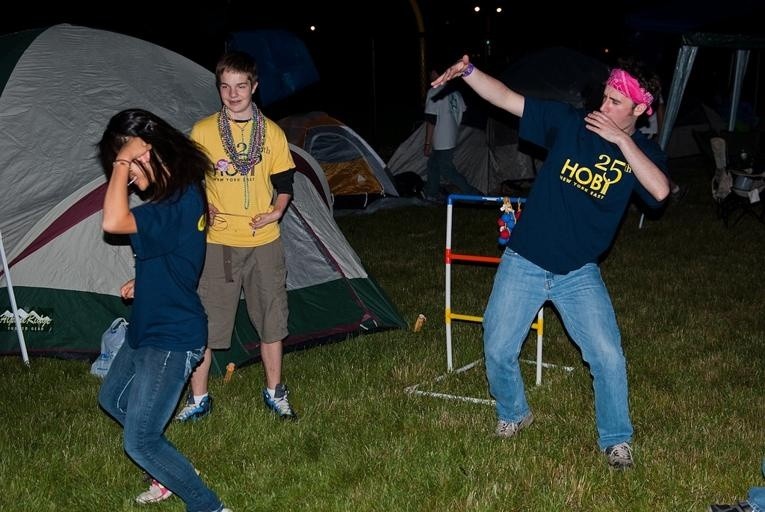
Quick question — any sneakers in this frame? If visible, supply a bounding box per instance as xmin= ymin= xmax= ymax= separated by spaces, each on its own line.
xmin=135 ymin=462 xmax=201 ymax=504
xmin=600 ymin=441 xmax=634 ymax=469
xmin=492 ymin=411 xmax=533 ymax=438
xmin=262 ymin=383 xmax=297 ymax=419
xmin=174 ymin=390 xmax=212 ymax=424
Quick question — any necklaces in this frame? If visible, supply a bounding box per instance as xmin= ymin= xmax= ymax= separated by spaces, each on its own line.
xmin=225 ymin=110 xmax=254 ymax=148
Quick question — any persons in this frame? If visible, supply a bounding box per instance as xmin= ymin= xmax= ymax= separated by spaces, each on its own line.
xmin=94 ymin=108 xmax=230 ymax=511
xmin=429 ymin=54 xmax=671 ymax=470
xmin=637 ymin=90 xmax=681 ymax=195
xmin=420 ymin=63 xmax=489 ymax=209
xmin=176 ymin=51 xmax=299 ymax=424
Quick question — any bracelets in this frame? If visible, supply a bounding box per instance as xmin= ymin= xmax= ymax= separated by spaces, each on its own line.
xmin=110 ymin=159 xmax=131 ymax=167
xmin=424 ymin=142 xmax=431 ymax=146
xmin=460 ymin=62 xmax=474 ymax=79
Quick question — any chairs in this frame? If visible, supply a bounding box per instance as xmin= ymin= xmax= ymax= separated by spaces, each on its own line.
xmin=690 ymin=128 xmax=765 ymax=228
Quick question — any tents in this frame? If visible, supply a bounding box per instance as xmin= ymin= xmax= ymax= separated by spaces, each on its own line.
xmin=658 ymin=31 xmax=765 ymax=139
xmin=270 ymin=110 xmax=400 ymax=212
xmin=385 ymin=112 xmax=505 ymax=197
xmin=488 ymin=44 xmax=712 ymax=184
xmin=213 ymin=28 xmax=324 ymax=111
xmin=0 ymin=22 xmax=413 ymax=375
